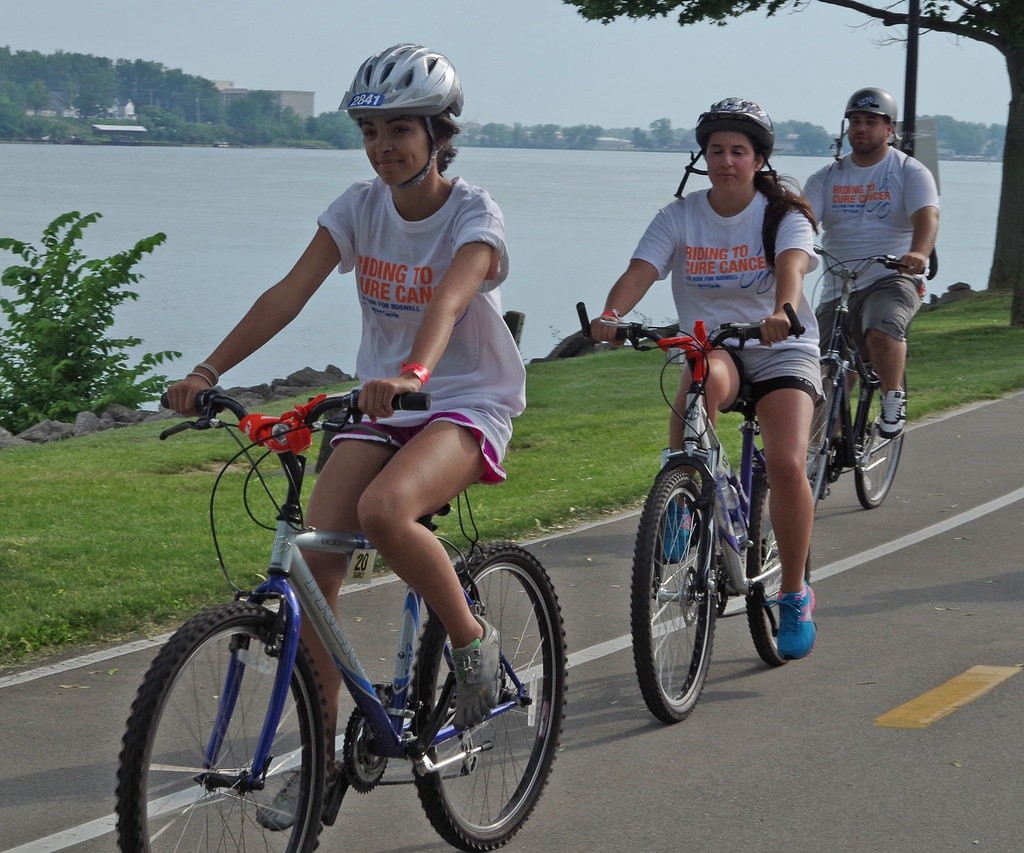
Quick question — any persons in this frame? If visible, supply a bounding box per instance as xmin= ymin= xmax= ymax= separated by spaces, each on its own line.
xmin=166 ymin=43 xmax=526 ymax=831
xmin=591 ymin=97 xmax=824 ymax=661
xmin=798 ymin=88 xmax=940 ymax=487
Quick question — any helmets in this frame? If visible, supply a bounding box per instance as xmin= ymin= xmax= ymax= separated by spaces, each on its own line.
xmin=339 ymin=41 xmax=464 ymax=117
xmin=695 ymin=96 xmax=774 ymax=142
xmin=844 ymin=86 xmax=897 ymax=123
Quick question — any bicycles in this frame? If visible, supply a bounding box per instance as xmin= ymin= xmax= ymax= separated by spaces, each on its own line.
xmin=576 ymin=302 xmax=818 ymax=725
xmin=115 ymin=389 xmax=569 ymax=852
xmin=810 ymin=244 xmax=938 ymax=510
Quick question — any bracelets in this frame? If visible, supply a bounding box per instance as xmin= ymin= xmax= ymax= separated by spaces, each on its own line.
xmin=187 ymin=363 xmax=218 ymax=388
xmin=399 ymin=361 xmax=430 ymax=385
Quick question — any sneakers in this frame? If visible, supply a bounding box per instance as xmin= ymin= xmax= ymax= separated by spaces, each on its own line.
xmin=807 ymin=475 xmax=816 ymax=489
xmin=255 ymin=755 xmax=343 ymax=832
xmin=453 ymin=615 xmax=500 ymax=732
xmin=663 ymin=498 xmax=691 ymax=564
xmin=763 ymin=579 xmax=816 ymax=661
xmin=879 ymin=386 xmax=909 ymax=439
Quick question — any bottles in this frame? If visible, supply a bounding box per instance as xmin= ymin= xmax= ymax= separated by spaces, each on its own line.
xmin=716 ymin=466 xmax=749 ymax=549
